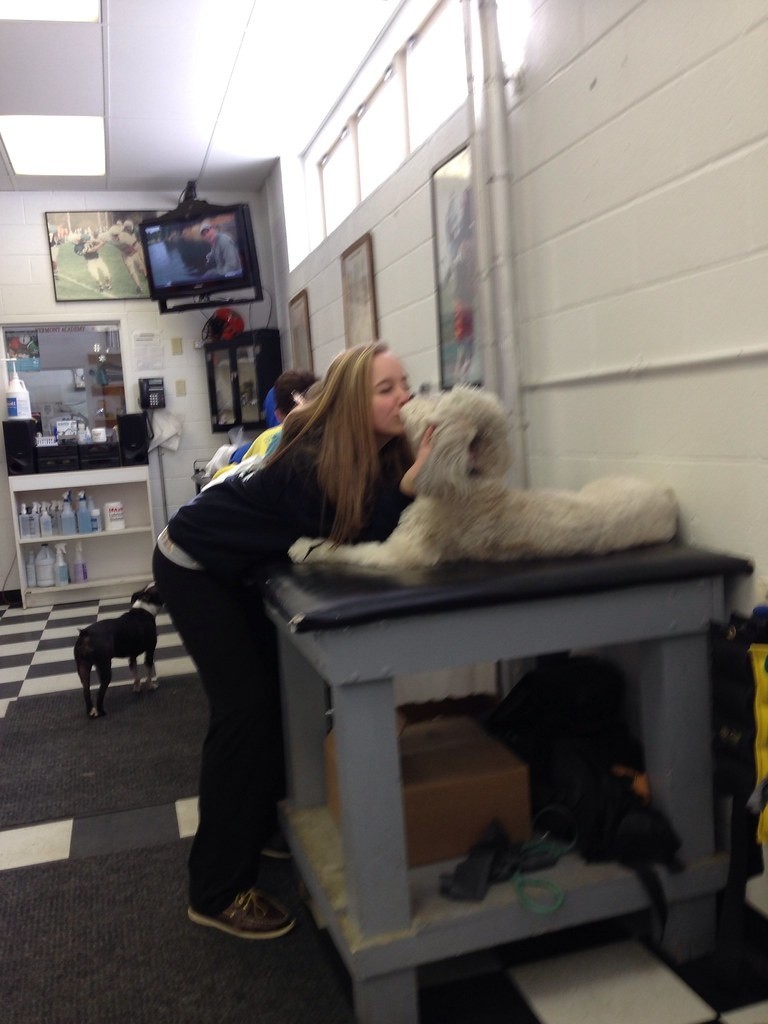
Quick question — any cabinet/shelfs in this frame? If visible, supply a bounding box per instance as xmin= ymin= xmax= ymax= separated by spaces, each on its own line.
xmin=263 ymin=541 xmax=756 ymax=1024
xmin=8 ymin=465 xmax=156 ymax=609
xmin=204 ymin=328 xmax=281 ymax=435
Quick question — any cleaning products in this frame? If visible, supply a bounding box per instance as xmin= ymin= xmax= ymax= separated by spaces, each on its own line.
xmin=19 ymin=488 xmax=125 ymax=586
xmin=4 ymin=358 xmax=31 ymax=419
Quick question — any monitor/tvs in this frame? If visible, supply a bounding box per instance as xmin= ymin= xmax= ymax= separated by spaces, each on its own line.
xmin=139 ymin=203 xmax=264 ymax=314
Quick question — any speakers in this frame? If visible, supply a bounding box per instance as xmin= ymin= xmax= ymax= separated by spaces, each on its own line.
xmin=2 ymin=420 xmax=37 ymax=476
xmin=117 ymin=413 xmax=150 ymax=465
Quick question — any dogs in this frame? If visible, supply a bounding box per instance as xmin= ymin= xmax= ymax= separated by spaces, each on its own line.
xmin=72 ymin=579 xmax=169 ymax=721
xmin=286 ymin=380 xmax=681 ymax=586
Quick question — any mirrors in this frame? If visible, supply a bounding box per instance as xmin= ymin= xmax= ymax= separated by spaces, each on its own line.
xmin=0 ymin=312 xmax=135 ymax=436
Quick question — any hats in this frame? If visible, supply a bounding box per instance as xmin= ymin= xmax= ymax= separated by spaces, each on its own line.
xmin=198 ymin=222 xmax=211 ymax=235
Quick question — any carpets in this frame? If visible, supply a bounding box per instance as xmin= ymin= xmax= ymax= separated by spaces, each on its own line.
xmin=0 ymin=673 xmax=543 ymax=1023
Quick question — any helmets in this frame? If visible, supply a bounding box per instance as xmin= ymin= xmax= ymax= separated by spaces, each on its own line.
xmin=208 ymin=309 xmax=244 ymax=341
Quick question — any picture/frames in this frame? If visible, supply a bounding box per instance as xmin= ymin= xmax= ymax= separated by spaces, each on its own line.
xmin=428 ymin=135 xmax=483 ymax=392
xmin=287 ymin=289 xmax=313 ymax=371
xmin=45 ymin=210 xmax=172 ymax=302
xmin=339 ymin=232 xmax=379 ymax=349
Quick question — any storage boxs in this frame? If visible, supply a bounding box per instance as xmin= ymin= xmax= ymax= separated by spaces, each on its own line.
xmin=320 ymin=722 xmax=532 ymax=868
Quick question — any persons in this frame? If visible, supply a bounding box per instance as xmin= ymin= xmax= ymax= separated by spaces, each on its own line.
xmin=152 ymin=342 xmax=436 ymax=939
xmin=200 ymin=224 xmax=241 ymax=279
xmin=49 ymin=220 xmax=147 ymax=294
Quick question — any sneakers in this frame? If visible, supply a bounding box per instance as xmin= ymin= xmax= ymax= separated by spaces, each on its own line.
xmin=187 ymin=883 xmax=296 ymax=940
xmin=262 ymin=834 xmax=293 ymax=860
